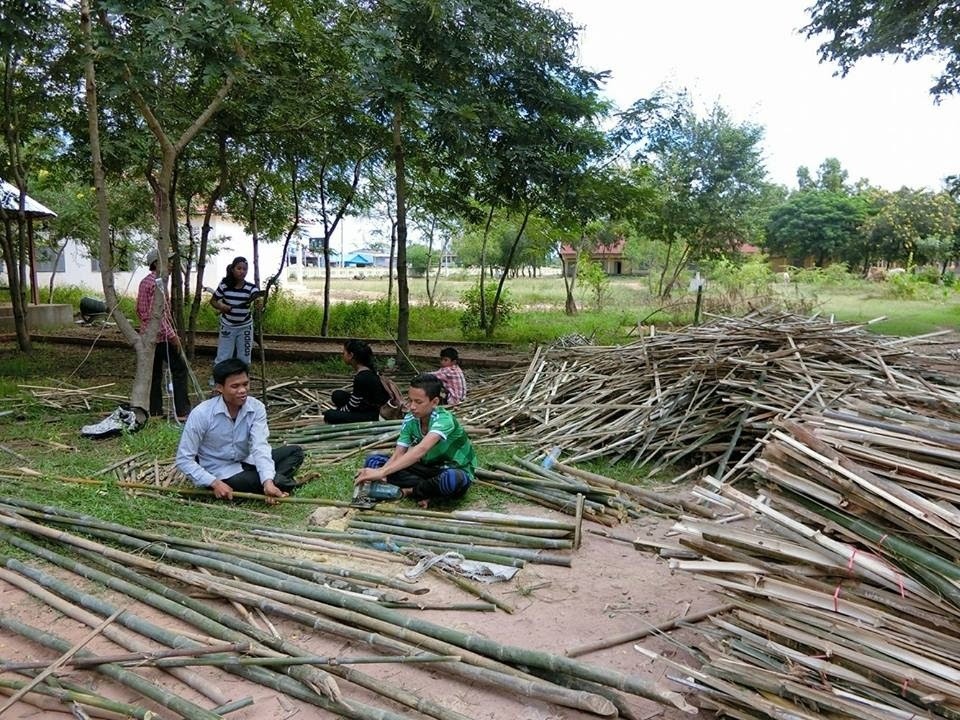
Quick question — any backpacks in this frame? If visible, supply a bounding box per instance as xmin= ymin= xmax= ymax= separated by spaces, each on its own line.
xmin=374 ymin=372 xmax=409 ymax=420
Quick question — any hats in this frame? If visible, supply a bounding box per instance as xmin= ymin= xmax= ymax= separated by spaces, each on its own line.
xmin=147 ymin=250 xmax=178 ymax=269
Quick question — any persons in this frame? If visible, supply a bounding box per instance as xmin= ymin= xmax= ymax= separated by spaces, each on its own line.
xmin=176 ymin=357 xmax=304 ymax=505
xmin=324 ymin=339 xmax=387 ymax=424
xmin=208 ymin=256 xmax=261 ymax=385
xmin=354 ymin=372 xmax=478 ymax=509
xmin=137 ymin=250 xmax=193 ymax=422
xmin=421 ymin=348 xmax=467 ymax=406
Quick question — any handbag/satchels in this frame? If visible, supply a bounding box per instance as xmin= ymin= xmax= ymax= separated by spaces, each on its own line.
xmin=81 ymin=404 xmax=148 ymax=436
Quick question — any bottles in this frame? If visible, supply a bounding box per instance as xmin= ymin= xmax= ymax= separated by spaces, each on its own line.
xmin=541 ymin=447 xmax=561 ymax=469
xmin=209 ymin=375 xmax=214 ymax=386
